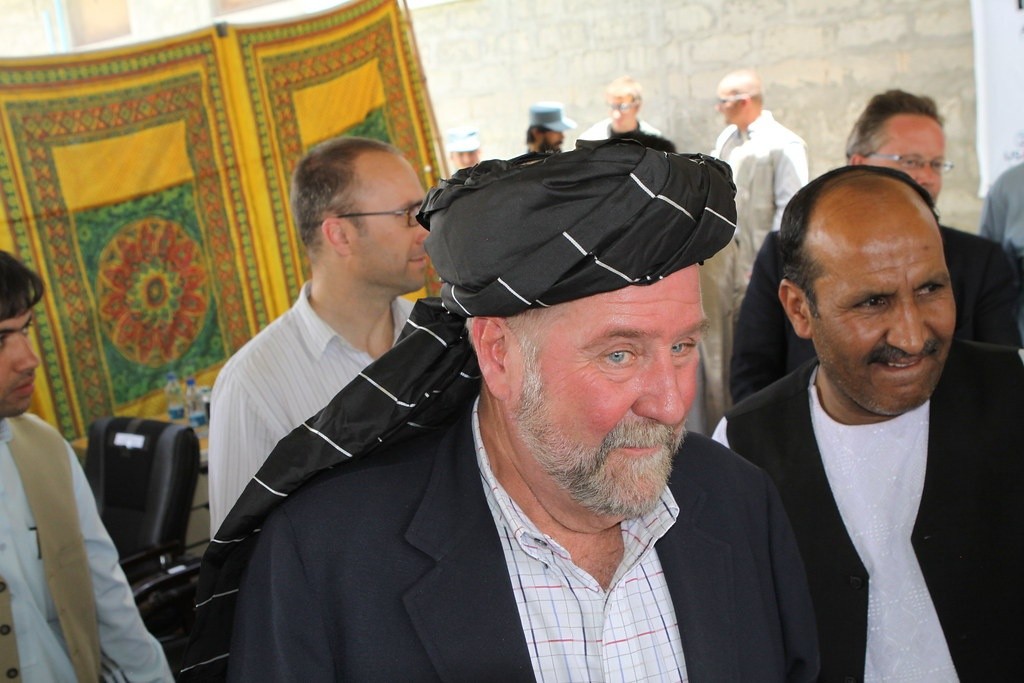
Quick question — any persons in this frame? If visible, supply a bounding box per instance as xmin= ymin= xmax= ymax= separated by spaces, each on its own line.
xmin=978 ymin=163 xmax=1024 ymax=269
xmin=0 ymin=251 xmax=175 ymax=683
xmin=575 ymin=81 xmax=662 ymax=141
xmin=445 ymin=127 xmax=481 ymax=171
xmin=711 ymin=165 xmax=1024 ymax=683
xmin=209 ymin=135 xmax=430 ymax=545
xmin=526 ymin=104 xmax=575 ymax=154
xmin=699 ymin=70 xmax=808 ymax=432
xmin=182 ymin=140 xmax=821 ymax=683
xmin=729 ymin=90 xmax=1024 ymax=405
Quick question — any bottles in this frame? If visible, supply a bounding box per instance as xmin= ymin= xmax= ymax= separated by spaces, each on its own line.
xmin=164 ymin=365 xmax=212 ymax=439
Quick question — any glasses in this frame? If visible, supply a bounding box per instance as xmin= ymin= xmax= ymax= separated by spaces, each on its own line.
xmin=869 ymin=152 xmax=954 ymax=176
xmin=313 ymin=201 xmax=421 ymax=228
xmin=714 ymin=94 xmax=748 ymax=105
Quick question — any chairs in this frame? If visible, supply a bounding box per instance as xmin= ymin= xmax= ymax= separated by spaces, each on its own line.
xmin=82 ymin=415 xmax=210 ymax=674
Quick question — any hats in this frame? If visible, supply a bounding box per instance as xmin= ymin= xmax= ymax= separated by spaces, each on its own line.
xmin=415 ymin=138 xmax=738 ymax=319
xmin=529 ymin=103 xmax=575 ymax=131
xmin=442 ymin=118 xmax=479 ymax=151
